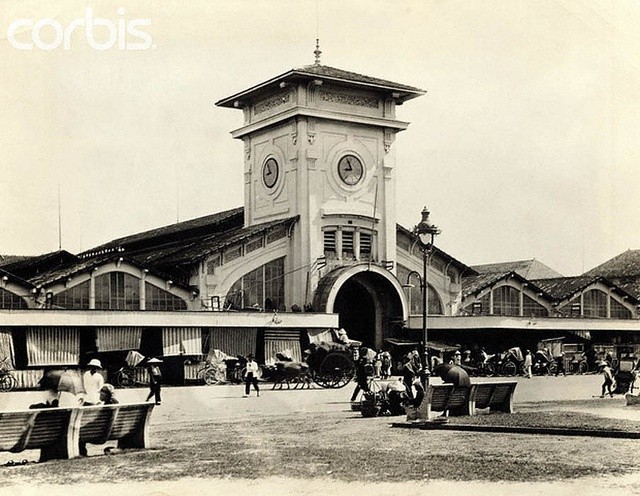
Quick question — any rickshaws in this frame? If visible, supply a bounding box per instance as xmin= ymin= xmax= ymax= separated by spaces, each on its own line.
xmin=196 ymin=348 xmax=239 ymax=385
xmin=112 ymin=350 xmax=146 ymax=388
xmin=606 ymin=357 xmax=637 ymax=397
xmin=536 ymin=350 xmax=558 ymax=376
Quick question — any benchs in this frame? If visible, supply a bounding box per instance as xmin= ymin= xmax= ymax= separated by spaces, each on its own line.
xmin=426 ymin=380 xmax=518 ymax=420
xmin=0 ymin=402 xmax=155 ymax=462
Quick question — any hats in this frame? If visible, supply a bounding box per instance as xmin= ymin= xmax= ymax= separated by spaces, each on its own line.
xmin=148 ymin=358 xmax=163 ymax=362
xmin=598 ymin=361 xmax=608 ymax=367
xmin=246 ymin=353 xmax=255 ymax=357
xmin=102 ymin=383 xmax=114 ymax=394
xmin=87 ymin=358 xmax=102 ymax=368
xmin=388 ymin=382 xmax=406 ymax=392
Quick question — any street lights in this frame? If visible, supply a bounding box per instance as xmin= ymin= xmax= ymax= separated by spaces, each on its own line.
xmin=412 ymin=205 xmax=441 ymax=394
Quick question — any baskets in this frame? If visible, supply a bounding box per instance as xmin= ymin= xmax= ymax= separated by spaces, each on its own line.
xmin=362 ymin=401 xmax=376 ymax=406
xmin=350 ymin=401 xmax=362 ymax=411
xmin=363 ymin=393 xmax=379 ymax=400
xmin=361 ymin=406 xmax=381 ymax=417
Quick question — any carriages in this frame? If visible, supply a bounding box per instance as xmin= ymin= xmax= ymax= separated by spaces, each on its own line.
xmin=261 ymin=339 xmax=362 ymax=390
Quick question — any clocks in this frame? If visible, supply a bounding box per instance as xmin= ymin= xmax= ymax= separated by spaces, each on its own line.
xmin=263 ymin=158 xmax=278 ymax=188
xmin=338 ymin=155 xmax=363 ymax=185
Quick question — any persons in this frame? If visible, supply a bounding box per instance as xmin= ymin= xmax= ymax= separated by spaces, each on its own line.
xmin=83 ymin=359 xmax=103 ymax=404
xmin=510 ymin=346 xmax=524 ymax=376
xmin=553 ymin=353 xmax=566 ymax=376
xmin=350 ymin=358 xmax=371 ymax=401
xmin=523 ymin=350 xmax=532 ymax=378
xmin=80 ymin=385 xmax=119 ymax=455
xmin=598 ymin=360 xmax=614 ymax=399
xmin=403 ymin=357 xmax=416 ymax=397
xmin=241 ymin=354 xmax=259 ymax=394
xmin=406 ymin=348 xmax=463 ymax=373
xmin=438 ymin=368 xmax=459 ymax=400
xmin=145 ymin=357 xmax=165 ymax=406
xmin=481 ymin=347 xmax=492 ymax=371
xmin=403 ymin=383 xmax=424 ymax=408
xmin=360 ymin=351 xmax=392 ymax=380
